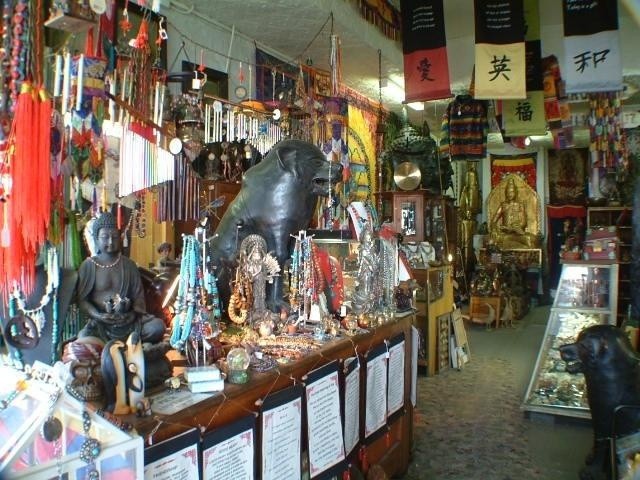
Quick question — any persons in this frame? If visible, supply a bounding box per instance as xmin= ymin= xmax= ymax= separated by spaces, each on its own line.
xmin=462 ymin=161 xmax=484 ymax=221
xmin=74 ymin=213 xmax=167 ymax=343
xmin=237 ymin=235 xmax=273 ymax=324
xmin=488 ymin=180 xmax=537 ymax=248
xmin=355 ymin=230 xmax=378 ymax=296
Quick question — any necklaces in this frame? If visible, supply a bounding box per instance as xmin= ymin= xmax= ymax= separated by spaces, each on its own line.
xmin=162 ymin=235 xmax=226 ymax=363
xmin=347 ymin=202 xmax=371 ymax=237
xmin=285 ymin=235 xmax=332 ymax=322
xmin=86 ymin=252 xmax=127 ymax=271
xmin=238 ymin=328 xmax=322 ymax=363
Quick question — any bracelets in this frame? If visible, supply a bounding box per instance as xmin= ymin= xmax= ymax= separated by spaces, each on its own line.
xmin=229 ymin=266 xmax=255 ymax=326
